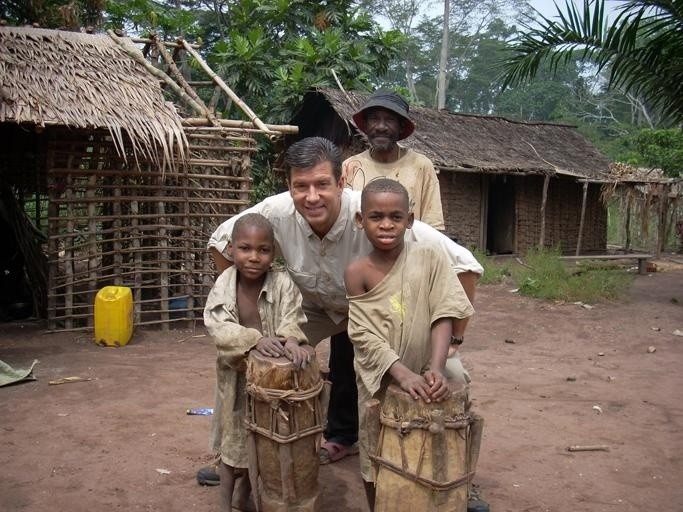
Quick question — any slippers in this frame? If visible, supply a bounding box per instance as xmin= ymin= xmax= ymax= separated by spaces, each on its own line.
xmin=319 ymin=441 xmax=359 ymax=465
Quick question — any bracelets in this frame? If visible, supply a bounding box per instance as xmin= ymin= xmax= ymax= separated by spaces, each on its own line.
xmin=450 ymin=335 xmax=464 ymax=346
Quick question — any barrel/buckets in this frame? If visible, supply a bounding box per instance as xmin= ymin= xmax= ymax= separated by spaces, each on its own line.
xmin=94 ymin=286 xmax=134 ymax=346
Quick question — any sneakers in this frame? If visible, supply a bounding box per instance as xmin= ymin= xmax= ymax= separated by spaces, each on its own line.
xmin=197 ymin=462 xmax=242 ymax=486
xmin=467 ymin=490 xmax=489 ymax=512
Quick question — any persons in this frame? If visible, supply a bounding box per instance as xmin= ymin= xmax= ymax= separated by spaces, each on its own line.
xmin=341 ymin=177 xmax=475 ymax=511
xmin=206 ymin=214 xmax=308 ymax=512
xmin=197 ymin=136 xmax=491 ymax=510
xmin=311 ymin=90 xmax=446 ymax=465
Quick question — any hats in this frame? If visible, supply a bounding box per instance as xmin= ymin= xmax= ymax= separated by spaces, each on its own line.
xmin=351 ymin=89 xmax=415 ymax=141
